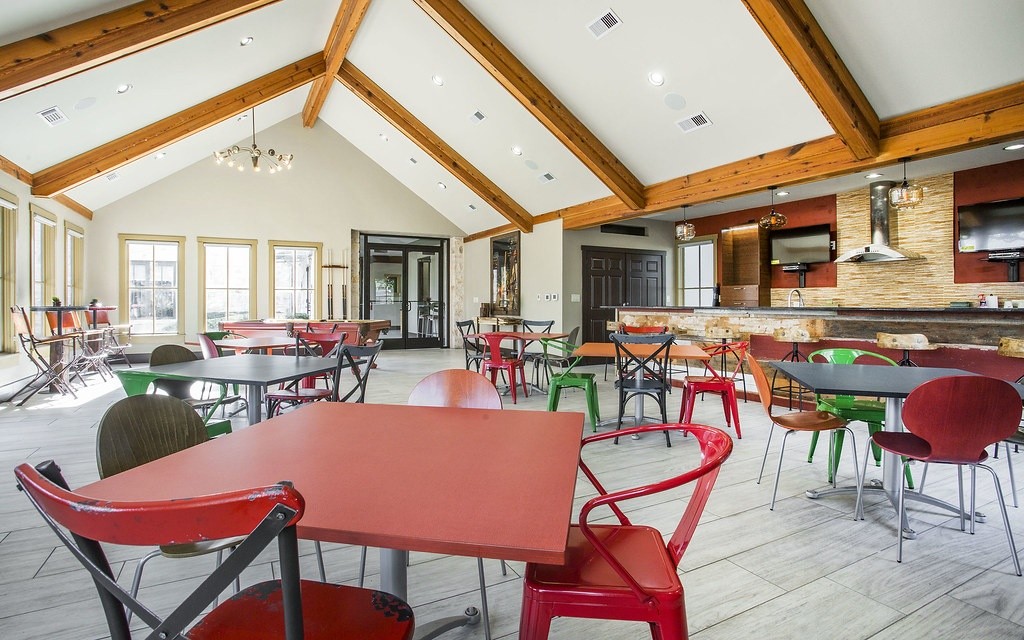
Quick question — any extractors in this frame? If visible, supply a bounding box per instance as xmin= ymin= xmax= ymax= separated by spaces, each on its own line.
xmin=834 ymin=180 xmax=928 ymax=264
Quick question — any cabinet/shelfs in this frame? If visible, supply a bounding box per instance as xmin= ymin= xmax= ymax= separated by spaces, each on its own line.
xmin=720 ymin=286 xmax=770 ymax=307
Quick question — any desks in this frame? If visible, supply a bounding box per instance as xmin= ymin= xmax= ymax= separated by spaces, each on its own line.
xmin=29 ymin=306 xmax=89 ymax=394
xmin=88 ymin=307 xmax=116 ymax=371
xmin=70 ymin=403 xmax=586 ymax=640
xmin=130 ymin=354 xmax=367 ymax=425
xmin=770 ymin=363 xmax=987 ymax=540
xmin=572 ymin=343 xmax=711 ymax=440
xmin=185 ymin=336 xmax=317 ymax=355
xmin=465 ymin=332 xmax=568 ymax=396
xmin=218 ymin=319 xmax=391 ymax=375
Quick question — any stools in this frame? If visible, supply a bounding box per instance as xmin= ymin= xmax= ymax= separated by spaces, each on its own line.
xmin=701 ymin=327 xmax=748 ymax=403
xmin=653 ymin=324 xmax=690 ymax=394
xmin=998 ymin=336 xmax=1024 ymax=385
xmin=875 ymin=332 xmax=937 ymax=366
xmin=605 ymin=321 xmax=636 ymax=381
xmin=417 ymin=301 xmax=439 ymax=337
xmin=771 ymin=328 xmax=823 ymax=412
xmin=6 ymin=305 xmax=132 ymax=407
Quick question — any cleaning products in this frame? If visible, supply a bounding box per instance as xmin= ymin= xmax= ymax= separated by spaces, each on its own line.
xmin=978 ymin=294 xmax=986 ymax=307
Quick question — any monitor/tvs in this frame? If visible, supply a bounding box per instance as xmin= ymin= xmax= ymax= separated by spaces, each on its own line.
xmin=956 ymin=197 xmax=1024 ymax=253
xmin=769 ymin=222 xmax=832 ymax=264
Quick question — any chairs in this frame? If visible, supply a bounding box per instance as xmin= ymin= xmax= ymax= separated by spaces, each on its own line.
xmin=11 ymin=320 xmax=1024 ymax=640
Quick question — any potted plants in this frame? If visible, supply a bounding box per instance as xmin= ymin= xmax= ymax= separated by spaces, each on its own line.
xmin=52 ymin=296 xmax=61 ymax=307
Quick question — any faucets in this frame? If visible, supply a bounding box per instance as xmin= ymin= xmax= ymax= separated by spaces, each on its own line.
xmin=788 ymin=289 xmax=801 ymax=307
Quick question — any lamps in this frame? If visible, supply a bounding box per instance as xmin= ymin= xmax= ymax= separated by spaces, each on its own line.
xmin=212 ymin=107 xmax=293 ymax=173
xmin=759 ymin=185 xmax=788 ymax=230
xmin=676 ymin=205 xmax=695 ymax=240
xmin=887 ymin=156 xmax=924 ymax=210
